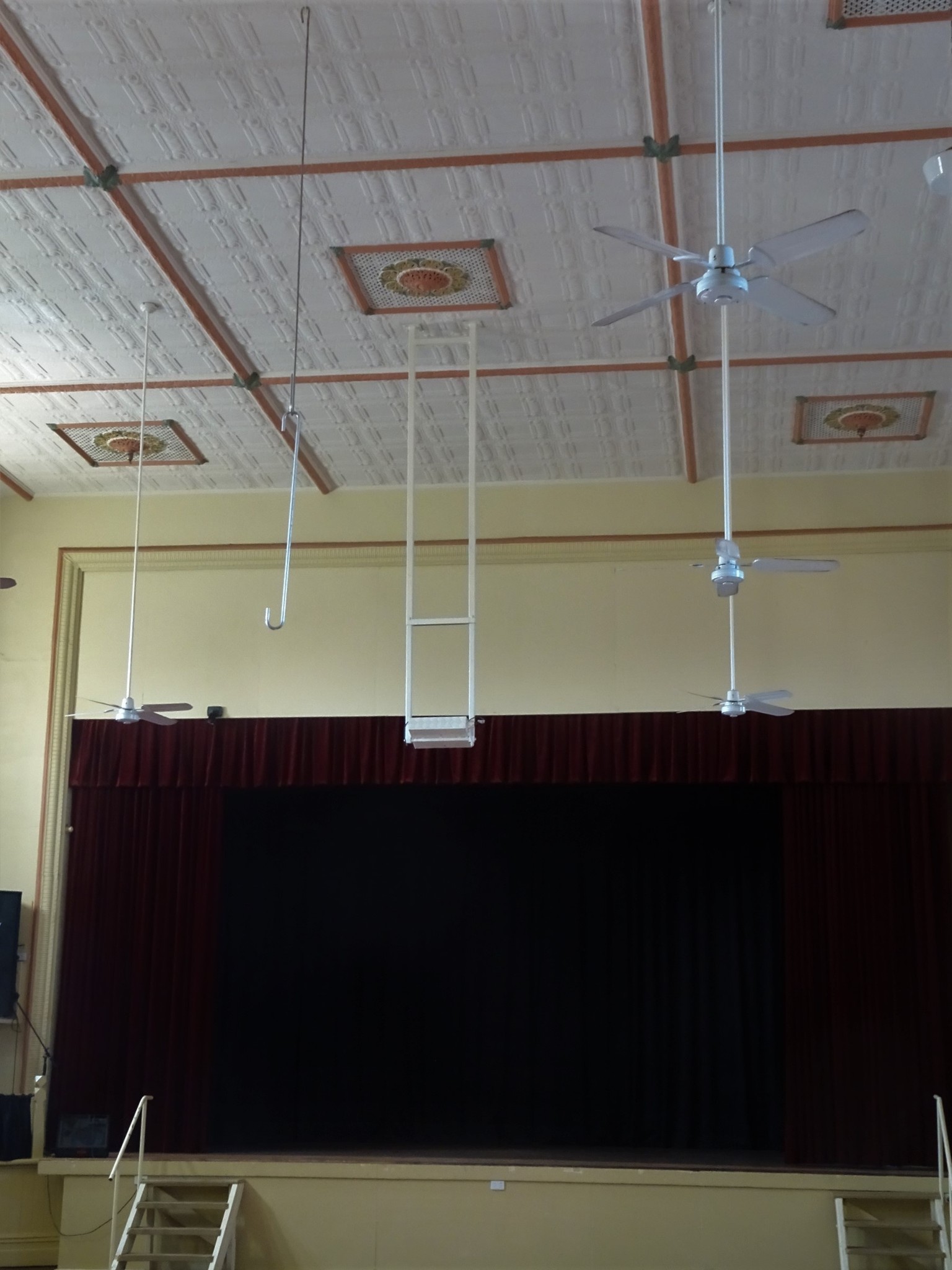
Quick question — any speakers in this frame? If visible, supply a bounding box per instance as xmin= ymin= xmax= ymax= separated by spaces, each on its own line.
xmin=0 ymin=891 xmax=22 ymax=1018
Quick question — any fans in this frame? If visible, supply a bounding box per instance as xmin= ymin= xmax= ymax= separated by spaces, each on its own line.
xmin=582 ymin=0 xmax=871 ymax=721
xmin=57 ymin=302 xmax=197 ymax=729
